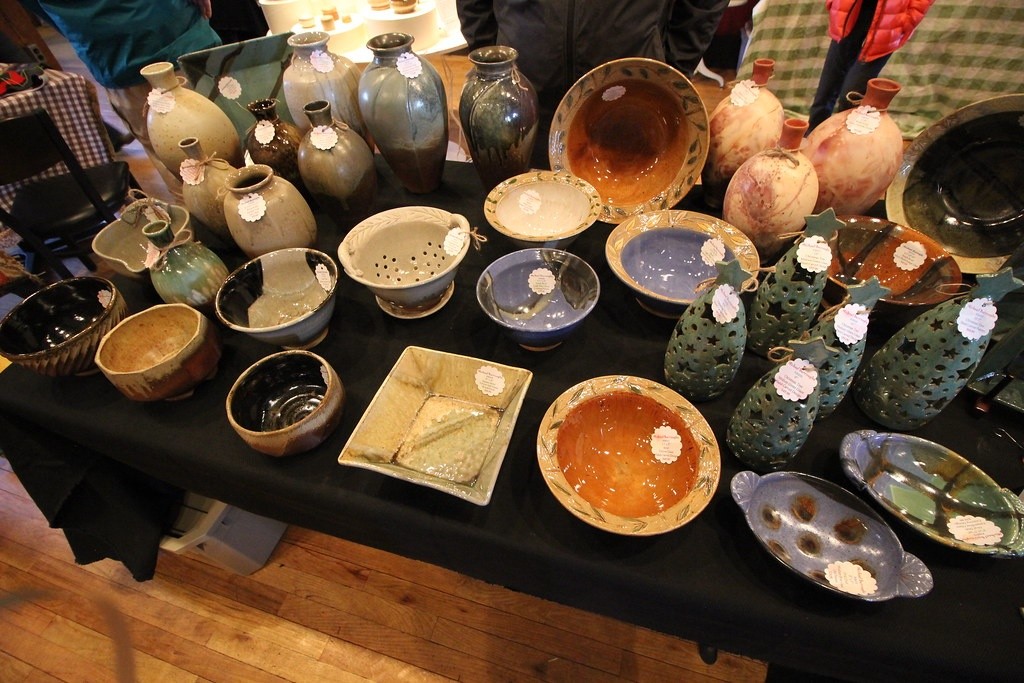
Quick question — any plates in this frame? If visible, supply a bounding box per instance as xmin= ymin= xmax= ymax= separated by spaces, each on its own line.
xmin=963 ymin=272 xmax=1024 ymax=413
xmin=177 ymin=31 xmax=296 ymax=166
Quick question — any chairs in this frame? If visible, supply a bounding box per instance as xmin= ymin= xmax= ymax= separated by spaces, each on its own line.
xmin=0 ymin=107 xmax=131 ymax=280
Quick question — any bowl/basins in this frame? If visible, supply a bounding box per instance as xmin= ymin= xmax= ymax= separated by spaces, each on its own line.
xmin=825 ymin=215 xmax=961 ymax=306
xmin=475 ymin=249 xmax=601 ymax=352
xmin=1 ymin=277 xmax=131 ymax=378
xmin=215 ymin=248 xmax=338 ymax=351
xmin=730 ymin=470 xmax=933 ymax=602
xmin=549 ymin=58 xmax=711 ymax=224
xmin=537 ymin=373 xmax=721 ymax=536
xmin=886 ymin=93 xmax=1024 ymax=275
xmin=338 ymin=346 xmax=533 ymax=506
xmin=338 ymin=206 xmax=471 ymax=318
xmin=226 ymin=349 xmax=346 ymax=457
xmin=91 ymin=196 xmax=191 ymax=281
xmin=484 ymin=171 xmax=601 ymax=248
xmin=95 ymin=302 xmax=223 ymax=403
xmin=840 ymin=430 xmax=1024 ymax=558
xmin=605 ymin=210 xmax=760 ymax=319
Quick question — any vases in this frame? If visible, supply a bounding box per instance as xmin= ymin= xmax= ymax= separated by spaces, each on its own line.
xmin=298 ymin=98 xmax=380 ymax=233
xmin=723 ymin=118 xmax=819 ymax=261
xmin=801 ymin=78 xmax=904 ymax=214
xmin=701 ymin=58 xmax=785 ymax=207
xmin=282 ymin=32 xmax=375 ymax=158
xmin=222 ymin=163 xmax=318 ymax=260
xmin=140 ymin=62 xmax=241 ymax=185
xmin=457 ymin=46 xmax=540 ymax=195
xmin=243 ymin=99 xmax=313 ymax=207
xmin=179 ymin=137 xmax=239 ymax=242
xmin=142 ymin=221 xmax=230 ymax=320
xmin=358 ymin=33 xmax=449 ymax=195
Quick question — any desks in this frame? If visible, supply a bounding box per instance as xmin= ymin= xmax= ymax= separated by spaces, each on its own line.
xmin=0 ymin=62 xmax=117 ymax=214
xmin=0 ymin=158 xmax=1024 ymax=683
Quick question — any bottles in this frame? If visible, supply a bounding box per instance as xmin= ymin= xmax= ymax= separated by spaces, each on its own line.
xmin=140 ymin=31 xmax=541 ymax=321
xmin=701 ymin=59 xmax=904 ymax=265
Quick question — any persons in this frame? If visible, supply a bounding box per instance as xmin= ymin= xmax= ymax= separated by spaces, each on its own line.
xmin=456 ymin=0 xmax=760 ymax=173
xmin=36 ymin=0 xmax=272 ymax=208
xmin=804 ymin=0 xmax=935 ymax=137
